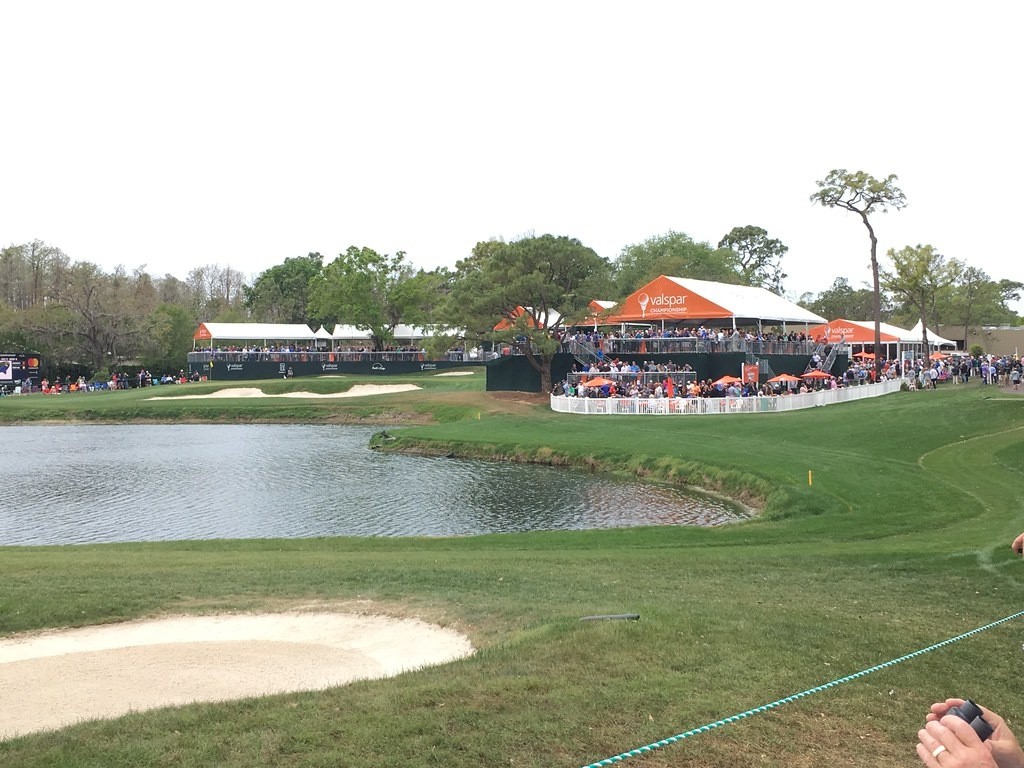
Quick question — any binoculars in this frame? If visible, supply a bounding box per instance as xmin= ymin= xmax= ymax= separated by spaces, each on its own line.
xmin=944 ymin=697 xmax=995 ymax=745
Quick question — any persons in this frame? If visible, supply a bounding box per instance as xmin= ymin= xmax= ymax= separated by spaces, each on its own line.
xmin=492 ymin=333 xmax=526 ymax=358
xmin=915 ymin=697 xmax=1024 ymax=768
xmin=1 ymin=370 xmax=208 ymax=395
xmin=553 ymin=325 xmax=1024 ymax=412
xmin=194 ymin=344 xmax=484 ymax=360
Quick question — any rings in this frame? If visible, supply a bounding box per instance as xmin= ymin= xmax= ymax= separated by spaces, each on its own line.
xmin=932 ymin=745 xmax=946 ymax=758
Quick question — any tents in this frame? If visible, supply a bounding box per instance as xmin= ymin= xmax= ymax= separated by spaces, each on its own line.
xmin=493 ymin=274 xmax=828 ymax=351
xmin=803 ymin=319 xmax=957 ymax=362
xmin=193 ymin=322 xmax=468 ymax=351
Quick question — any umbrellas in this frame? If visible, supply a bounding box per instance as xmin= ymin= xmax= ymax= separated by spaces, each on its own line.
xmin=852 ymin=351 xmax=867 ymax=356
xmin=865 ymin=353 xmax=883 ymax=359
xmin=802 ymin=370 xmax=830 ymax=381
xmin=766 ymin=374 xmax=803 ymax=385
xmin=712 ymin=375 xmax=742 ymax=386
xmin=583 ymin=377 xmax=614 ymax=386
xmin=925 ymin=352 xmax=950 ymax=360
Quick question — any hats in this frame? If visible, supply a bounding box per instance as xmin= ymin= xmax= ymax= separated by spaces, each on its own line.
xmin=588 ymin=332 xmax=590 ymax=333
xmin=791 ymin=331 xmax=794 ymax=333
xmin=609 ymin=332 xmax=612 ymax=334
xmin=577 ymin=331 xmax=580 ymax=333
xmin=679 ymin=381 xmax=682 ymax=383
xmin=775 ymin=384 xmax=779 ymax=387
xmin=694 ymin=381 xmax=697 ymax=384
xmin=654 ymin=383 xmax=659 ymax=386
xmin=650 ymin=380 xmax=653 ymax=382
